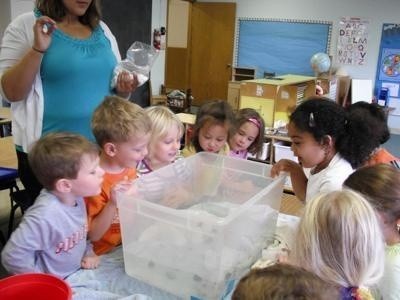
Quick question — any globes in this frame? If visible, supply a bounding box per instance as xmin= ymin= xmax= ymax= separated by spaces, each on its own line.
xmin=310 ymin=53 xmax=330 ymax=80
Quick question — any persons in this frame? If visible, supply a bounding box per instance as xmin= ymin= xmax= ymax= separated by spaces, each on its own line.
xmin=271 ymin=94 xmax=355 ymax=210
xmin=183 ymin=98 xmax=238 ymax=159
xmin=0 ymin=0 xmax=140 ymax=205
xmin=346 ymin=100 xmax=400 ymax=252
xmin=1 ymin=127 xmax=101 ymax=283
xmin=291 ymin=184 xmax=400 ymax=300
xmin=228 ymin=107 xmax=267 ymax=159
xmin=231 ymin=263 xmax=357 ymax=299
xmin=82 ymin=94 xmax=154 ymax=256
xmin=136 ymin=105 xmax=186 ymax=178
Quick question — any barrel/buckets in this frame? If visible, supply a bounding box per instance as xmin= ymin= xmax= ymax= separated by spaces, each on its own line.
xmin=0 ymin=272 xmax=72 ymax=300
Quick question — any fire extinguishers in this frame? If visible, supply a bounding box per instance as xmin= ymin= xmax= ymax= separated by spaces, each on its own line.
xmin=154 ymin=29 xmax=161 ymax=53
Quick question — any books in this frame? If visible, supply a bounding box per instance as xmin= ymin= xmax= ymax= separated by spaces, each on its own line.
xmin=296 ymin=84 xmax=308 ymax=106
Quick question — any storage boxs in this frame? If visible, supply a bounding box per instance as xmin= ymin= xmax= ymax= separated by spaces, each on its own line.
xmin=246 ymin=141 xmax=270 ymax=162
xmin=272 ymin=141 xmax=300 ymax=165
xmin=115 ymin=151 xmax=290 ymax=298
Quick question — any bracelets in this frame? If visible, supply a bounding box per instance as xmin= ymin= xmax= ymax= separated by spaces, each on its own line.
xmin=31 ymin=46 xmax=47 ymax=53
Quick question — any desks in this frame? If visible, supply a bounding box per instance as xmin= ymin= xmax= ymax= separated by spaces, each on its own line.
xmin=62 ymin=193 xmax=306 ymax=299
